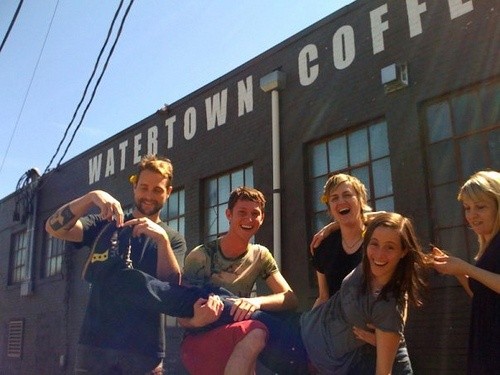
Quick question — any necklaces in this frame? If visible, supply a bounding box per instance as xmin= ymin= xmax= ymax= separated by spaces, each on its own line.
xmin=341 ymin=236 xmax=362 ymax=248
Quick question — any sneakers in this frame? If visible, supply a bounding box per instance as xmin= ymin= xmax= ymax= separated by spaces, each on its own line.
xmin=82 ymin=218 xmax=120 ymax=284
xmin=117 ymin=224 xmax=134 ymax=270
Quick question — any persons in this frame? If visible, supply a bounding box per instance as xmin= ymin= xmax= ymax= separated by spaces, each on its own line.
xmin=83 ymin=211 xmax=436 ymax=375
xmin=421 ymin=171 xmax=500 ymax=375
xmin=176 ymin=185 xmax=299 ymax=375
xmin=44 ymin=153 xmax=188 ymax=375
xmin=309 ymin=173 xmax=414 ymax=375
